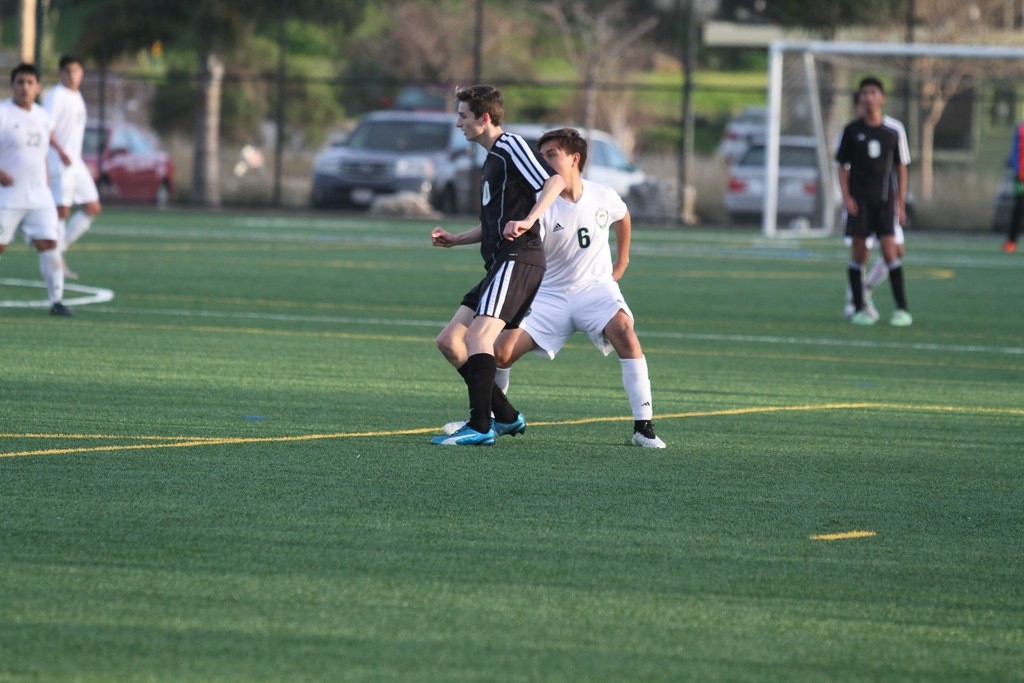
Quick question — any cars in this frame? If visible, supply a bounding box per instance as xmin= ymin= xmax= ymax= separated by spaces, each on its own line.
xmin=721 ymin=107 xmax=776 ymax=161
xmin=80 ymin=72 xmax=158 ymax=117
xmin=84 ymin=119 xmax=177 ymax=210
xmin=993 ymin=162 xmax=1017 ymax=234
xmin=500 ymin=123 xmax=662 ymax=218
xmin=311 ymin=111 xmax=487 ymax=214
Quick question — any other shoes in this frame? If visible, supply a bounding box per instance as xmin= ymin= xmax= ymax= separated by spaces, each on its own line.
xmin=890 ymin=309 xmax=912 ymax=327
xmin=851 ymin=310 xmax=875 ymax=326
xmin=50 ymin=302 xmax=72 ymax=317
xmin=866 ymin=299 xmax=880 ymax=321
xmin=845 ymin=304 xmax=856 ymax=319
xmin=1003 ymin=241 xmax=1017 ymax=252
xmin=63 ymin=260 xmax=79 ymax=280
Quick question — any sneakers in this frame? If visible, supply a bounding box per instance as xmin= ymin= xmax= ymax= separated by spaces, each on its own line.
xmin=494 ymin=410 xmax=526 ymax=437
xmin=442 ymin=420 xmax=470 ymax=435
xmin=631 ymin=431 xmax=666 ymax=449
xmin=430 ymin=417 xmax=496 ymax=446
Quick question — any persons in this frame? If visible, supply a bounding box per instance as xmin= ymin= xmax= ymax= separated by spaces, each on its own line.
xmin=0 ymin=63 xmax=75 ymax=317
xmin=1003 ymin=122 xmax=1024 ymax=254
xmin=834 ymin=77 xmax=913 ymax=327
xmin=430 ymin=86 xmax=565 ymax=446
xmin=832 ymin=93 xmax=912 ymax=320
xmin=446 ymin=128 xmax=666 ymax=449
xmin=41 ymin=55 xmax=101 ymax=280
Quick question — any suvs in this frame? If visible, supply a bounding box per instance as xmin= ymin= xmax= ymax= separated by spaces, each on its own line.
xmin=725 ymin=137 xmax=917 ymax=233
